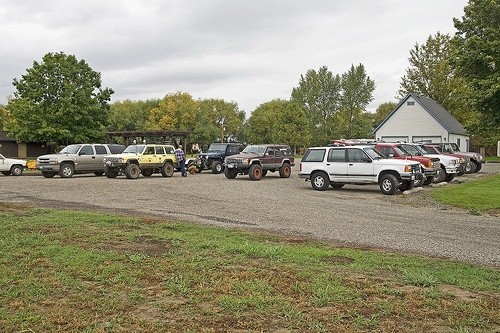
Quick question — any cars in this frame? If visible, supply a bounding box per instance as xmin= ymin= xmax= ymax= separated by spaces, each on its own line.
xmin=0 ymin=154 xmax=28 ymax=176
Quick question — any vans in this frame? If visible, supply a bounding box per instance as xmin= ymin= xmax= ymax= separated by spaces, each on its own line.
xmin=36 ymin=144 xmax=125 ymax=179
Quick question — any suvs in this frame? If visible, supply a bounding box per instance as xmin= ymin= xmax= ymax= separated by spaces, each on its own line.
xmin=435 ymin=143 xmax=486 ymax=173
xmin=359 ymin=142 xmax=440 ymax=185
xmin=298 ymin=145 xmax=425 ymax=196
xmin=102 ymin=145 xmax=179 ymax=179
xmin=222 ymin=144 xmax=295 ymax=181
xmin=409 ymin=143 xmax=461 ymax=183
xmin=197 ymin=143 xmax=239 ymax=174
xmin=423 ymin=145 xmax=470 ymax=177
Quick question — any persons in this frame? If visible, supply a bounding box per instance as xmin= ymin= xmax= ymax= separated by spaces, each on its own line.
xmin=174 ymin=145 xmax=187 ymax=177
